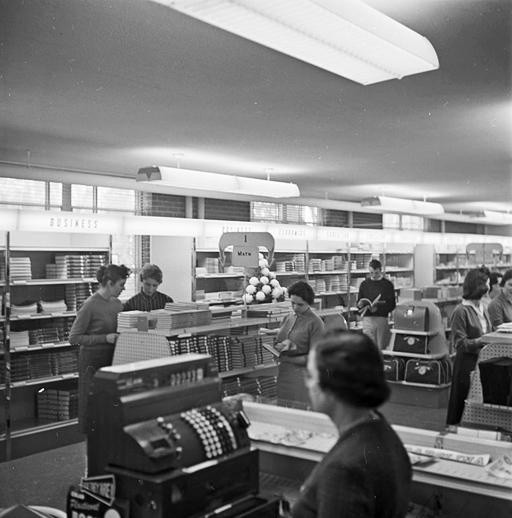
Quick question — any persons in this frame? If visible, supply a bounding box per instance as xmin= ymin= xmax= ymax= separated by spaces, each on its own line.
xmin=446 ymin=262 xmax=512 ymax=425
xmin=67 ymin=264 xmax=132 ymax=434
xmin=122 ymin=263 xmax=174 ymax=312
xmin=290 ymin=328 xmax=413 ymax=518
xmin=356 ymin=259 xmax=396 ymax=350
xmin=272 ymin=280 xmax=328 ymax=414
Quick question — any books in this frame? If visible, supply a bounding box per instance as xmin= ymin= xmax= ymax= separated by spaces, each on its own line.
xmin=194 ymin=253 xmax=305 ymax=300
xmin=117 ymin=303 xmax=306 ymax=406
xmin=0 ymin=251 xmax=108 ymax=419
xmin=308 ymin=251 xmax=512 ymax=304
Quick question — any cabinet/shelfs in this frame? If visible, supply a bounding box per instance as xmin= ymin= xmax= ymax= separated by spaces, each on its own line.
xmin=193 ymin=237 xmax=415 ymax=307
xmin=1 ymin=248 xmax=117 ymax=462
xmin=113 ymin=309 xmax=351 ymax=403
xmin=414 ymin=242 xmax=512 ymax=289
xmin=458 ymin=328 xmax=512 ymax=444
xmin=377 ymin=297 xmax=462 ymax=407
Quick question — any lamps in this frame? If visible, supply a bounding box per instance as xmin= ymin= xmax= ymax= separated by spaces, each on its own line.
xmin=152 ymin=0 xmax=439 ymax=86
xmin=468 ymin=206 xmax=512 ymax=222
xmin=137 ymin=162 xmax=300 ymax=202
xmin=361 ymin=197 xmax=446 ymax=215
